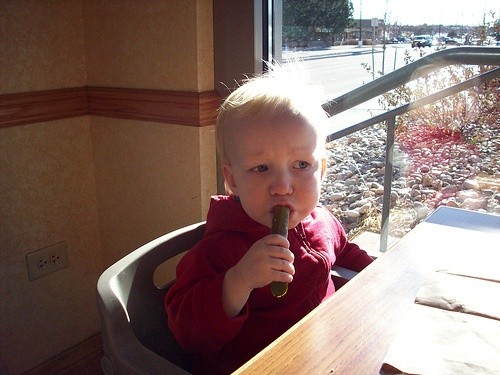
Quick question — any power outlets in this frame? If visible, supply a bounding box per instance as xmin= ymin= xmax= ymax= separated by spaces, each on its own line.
xmin=27 ymin=241 xmax=69 ymax=282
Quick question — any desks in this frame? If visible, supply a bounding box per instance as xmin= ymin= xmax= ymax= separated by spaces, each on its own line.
xmin=229 ymin=206 xmax=500 ymax=375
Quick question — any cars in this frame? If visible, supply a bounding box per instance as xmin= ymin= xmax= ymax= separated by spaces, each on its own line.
xmin=445 ymin=39 xmax=463 ymax=46
xmin=411 ymin=34 xmax=433 ymax=48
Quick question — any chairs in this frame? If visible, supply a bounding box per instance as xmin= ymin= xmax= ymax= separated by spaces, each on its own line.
xmin=95 ymin=220 xmax=359 ymax=375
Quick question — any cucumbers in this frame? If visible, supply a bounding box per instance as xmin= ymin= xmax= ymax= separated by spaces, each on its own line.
xmin=269 ymin=206 xmax=291 ymax=298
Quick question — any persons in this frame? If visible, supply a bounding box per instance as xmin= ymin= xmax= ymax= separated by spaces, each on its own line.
xmin=164 ymin=62 xmax=378 ymax=375
